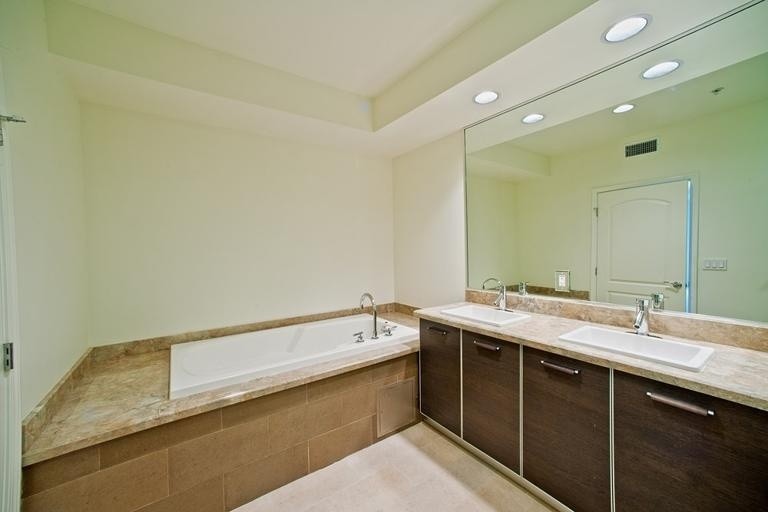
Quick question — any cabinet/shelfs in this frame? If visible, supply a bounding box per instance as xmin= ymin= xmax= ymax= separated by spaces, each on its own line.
xmin=518 ymin=342 xmax=612 ymax=512
xmin=460 ymin=328 xmax=521 ymax=486
xmin=417 ymin=315 xmax=463 ymax=445
xmin=609 ymin=367 xmax=767 ymax=512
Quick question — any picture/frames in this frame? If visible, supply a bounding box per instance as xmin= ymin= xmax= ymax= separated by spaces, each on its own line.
xmin=553 ymin=269 xmax=571 ymax=294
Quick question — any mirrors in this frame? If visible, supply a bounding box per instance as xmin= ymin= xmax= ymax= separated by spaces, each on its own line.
xmin=459 ymin=2 xmax=765 ymax=335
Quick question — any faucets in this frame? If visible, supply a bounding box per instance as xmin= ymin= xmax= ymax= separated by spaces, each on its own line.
xmin=634 ymin=298 xmax=650 ymax=334
xmin=482 ymin=278 xmax=506 ymax=310
xmin=651 ymin=294 xmax=670 ymax=310
xmin=359 ymin=291 xmax=378 ymax=341
xmin=519 ymin=281 xmax=530 ymax=296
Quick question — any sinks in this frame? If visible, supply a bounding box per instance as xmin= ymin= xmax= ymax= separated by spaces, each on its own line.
xmin=557 ymin=325 xmax=716 ymax=372
xmin=440 ymin=304 xmax=532 ymax=328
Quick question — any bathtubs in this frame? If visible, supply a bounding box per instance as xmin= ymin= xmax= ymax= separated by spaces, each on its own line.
xmin=169 ymin=312 xmax=419 ymax=398
